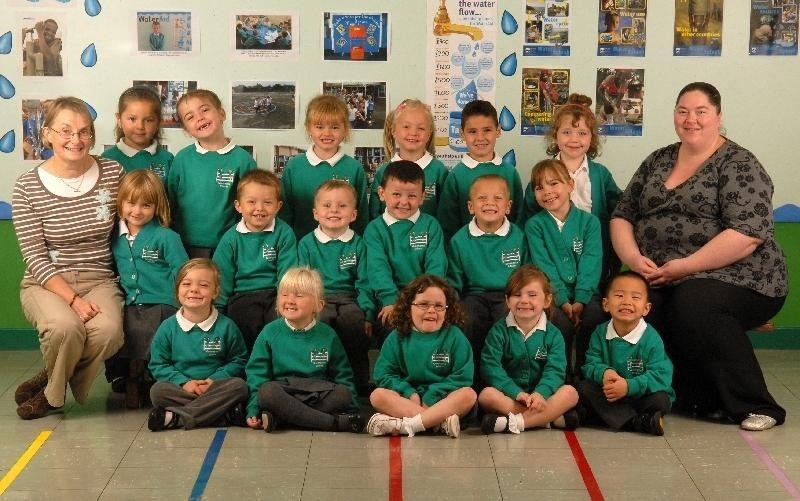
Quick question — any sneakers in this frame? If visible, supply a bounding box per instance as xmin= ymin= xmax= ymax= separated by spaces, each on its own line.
xmin=357 ymin=382 xmax=375 ymax=396
xmin=741 ymin=412 xmax=777 ymax=432
xmin=112 ymin=376 xmax=126 ymax=392
xmin=367 ymin=412 xmax=403 ymax=438
xmin=347 ymin=409 xmax=372 ymax=433
xmin=482 ymin=414 xmax=511 ymax=433
xmin=261 ymin=411 xmax=280 ymax=433
xmin=549 ymin=410 xmax=579 ymax=430
xmin=147 ymin=408 xmax=181 ymax=430
xmin=124 ymin=381 xmax=140 ymax=408
xmin=14 ymin=369 xmax=67 ymax=418
xmin=440 ymin=414 xmax=462 ymax=438
xmin=707 ymin=407 xmax=730 ymax=419
xmin=632 ymin=412 xmax=665 ymax=436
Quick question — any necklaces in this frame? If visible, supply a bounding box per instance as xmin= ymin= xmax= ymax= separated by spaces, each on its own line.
xmin=52 ymin=157 xmax=86 ymax=193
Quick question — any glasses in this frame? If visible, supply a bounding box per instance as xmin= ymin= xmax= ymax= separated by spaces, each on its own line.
xmin=411 ymin=301 xmax=450 ymax=311
xmin=48 ymin=126 xmax=94 ymax=141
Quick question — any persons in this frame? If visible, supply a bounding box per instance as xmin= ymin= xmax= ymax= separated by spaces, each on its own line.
xmin=608 ymin=82 xmax=788 ymax=430
xmin=336 ymin=89 xmax=375 ymax=127
xmin=254 ymin=96 xmax=273 ymax=114
xmin=576 ymin=270 xmax=674 ymax=435
xmin=366 ymin=274 xmax=478 ymax=436
xmin=295 ymin=180 xmax=375 ymax=372
xmin=244 ymin=266 xmax=367 ymax=434
xmin=23 ymin=18 xmax=63 ymax=76
xmin=278 ymin=95 xmax=371 ymax=240
xmin=523 ymin=158 xmax=605 ymax=372
xmin=11 ymin=97 xmax=128 ymax=420
xmin=211 ymin=169 xmax=298 ymax=357
xmin=147 ymin=22 xmax=167 ymax=51
xmin=369 ymin=98 xmax=448 ymax=220
xmin=101 ymin=85 xmax=174 ymax=178
xmin=435 ymin=99 xmax=523 ymax=242
xmin=166 ymin=90 xmax=258 ymax=259
xmin=446 ymin=173 xmax=529 ymax=359
xmin=597 ymin=71 xmax=628 ymax=124
xmin=147 ymin=257 xmax=247 ymax=430
xmin=236 ymin=15 xmax=291 ymax=49
xmin=104 ymin=169 xmax=189 ymax=407
xmin=363 ymin=160 xmax=447 ymax=341
xmin=598 ymin=12 xmax=622 ymax=45
xmin=478 ymin=265 xmax=579 ymax=434
xmin=523 ymin=93 xmax=623 ymax=274
